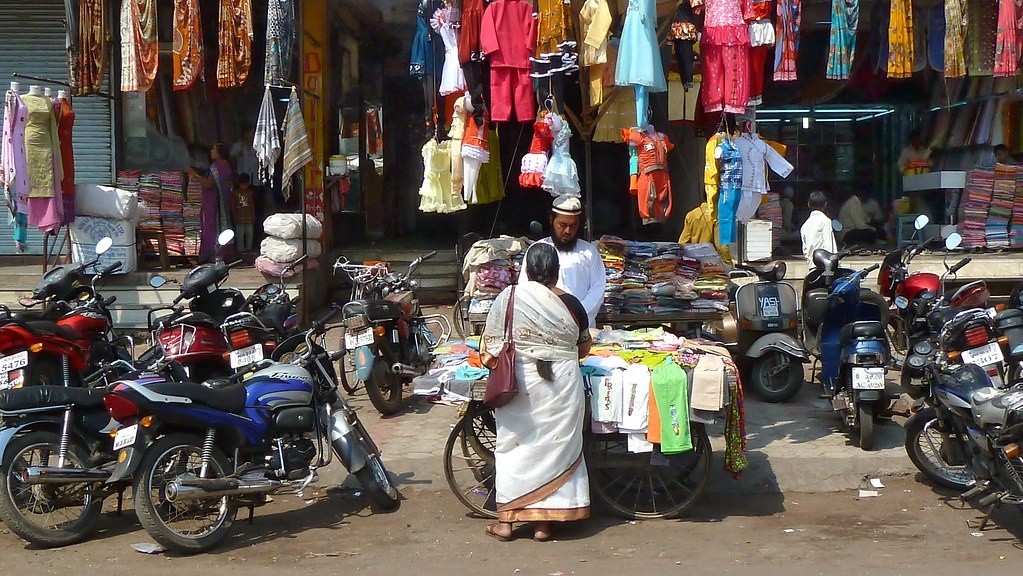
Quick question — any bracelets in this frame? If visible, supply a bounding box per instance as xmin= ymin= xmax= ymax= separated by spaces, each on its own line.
xmin=194 ymin=173 xmax=201 ymax=178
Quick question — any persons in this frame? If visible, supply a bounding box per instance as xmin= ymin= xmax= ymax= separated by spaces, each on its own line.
xmin=778 ymin=183 xmax=800 ymax=242
xmin=704 ymin=116 xmax=767 ymax=192
xmin=800 ymin=191 xmax=838 ymax=269
xmin=6 ymin=82 xmax=74 ymax=172
xmin=897 ymin=128 xmax=933 ymax=175
xmin=231 ymin=173 xmax=255 ymax=253
xmin=227 ymin=123 xmax=266 ymax=246
xmin=191 ymin=142 xmax=234 ymax=264
xmin=679 ymin=189 xmax=735 ymax=270
xmin=485 ymin=243 xmax=591 ymax=542
xmin=413 ymin=0 xmax=669 ymax=178
xmin=517 ymin=196 xmax=607 ymax=331
xmin=835 ymin=173 xmax=886 ymax=247
xmin=994 ymin=144 xmax=1019 ymax=165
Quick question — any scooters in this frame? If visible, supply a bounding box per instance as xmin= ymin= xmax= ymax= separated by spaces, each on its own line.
xmin=698 ymin=255 xmax=812 ymax=404
xmin=144 ymin=228 xmax=245 ymax=387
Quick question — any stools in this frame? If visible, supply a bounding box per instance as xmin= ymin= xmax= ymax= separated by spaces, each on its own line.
xmin=896 ymin=216 xmax=925 ymax=250
xmin=135 ymin=231 xmax=166 ymax=269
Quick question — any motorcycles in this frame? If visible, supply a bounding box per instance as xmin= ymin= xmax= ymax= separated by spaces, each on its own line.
xmin=217 ymin=252 xmax=311 ymax=386
xmin=102 ymin=304 xmax=399 ymax=553
xmin=0 ymin=305 xmax=190 ymax=547
xmin=799 ymin=240 xmax=897 ymax=452
xmin=890 ymin=297 xmax=1023 ymax=550
xmin=876 ymin=213 xmax=1023 ymax=390
xmin=0 ymin=236 xmax=136 ymax=399
xmin=341 ymin=251 xmax=453 ymax=416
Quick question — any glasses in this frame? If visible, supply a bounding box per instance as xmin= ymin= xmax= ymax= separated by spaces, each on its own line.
xmin=995 ymin=152 xmax=1003 ymax=156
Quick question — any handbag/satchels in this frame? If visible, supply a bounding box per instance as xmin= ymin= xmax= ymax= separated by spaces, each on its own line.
xmin=484 ymin=284 xmax=520 ymax=410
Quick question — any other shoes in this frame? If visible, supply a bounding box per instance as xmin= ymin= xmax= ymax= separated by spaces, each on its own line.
xmin=225 ymin=257 xmax=244 ymax=267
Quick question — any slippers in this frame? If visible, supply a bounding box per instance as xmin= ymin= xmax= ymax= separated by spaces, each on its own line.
xmin=859 ymin=248 xmax=871 ymax=255
xmin=484 ymin=522 xmax=511 ymax=542
xmin=532 ymin=526 xmax=550 ymax=541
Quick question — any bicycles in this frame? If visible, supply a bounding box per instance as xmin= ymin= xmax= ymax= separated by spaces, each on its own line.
xmin=330 ymin=252 xmax=404 ymax=393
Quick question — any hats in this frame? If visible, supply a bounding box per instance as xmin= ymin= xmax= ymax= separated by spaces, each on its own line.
xmin=783 ymin=186 xmax=793 ymax=196
xmin=552 ymin=195 xmax=582 ymax=216
